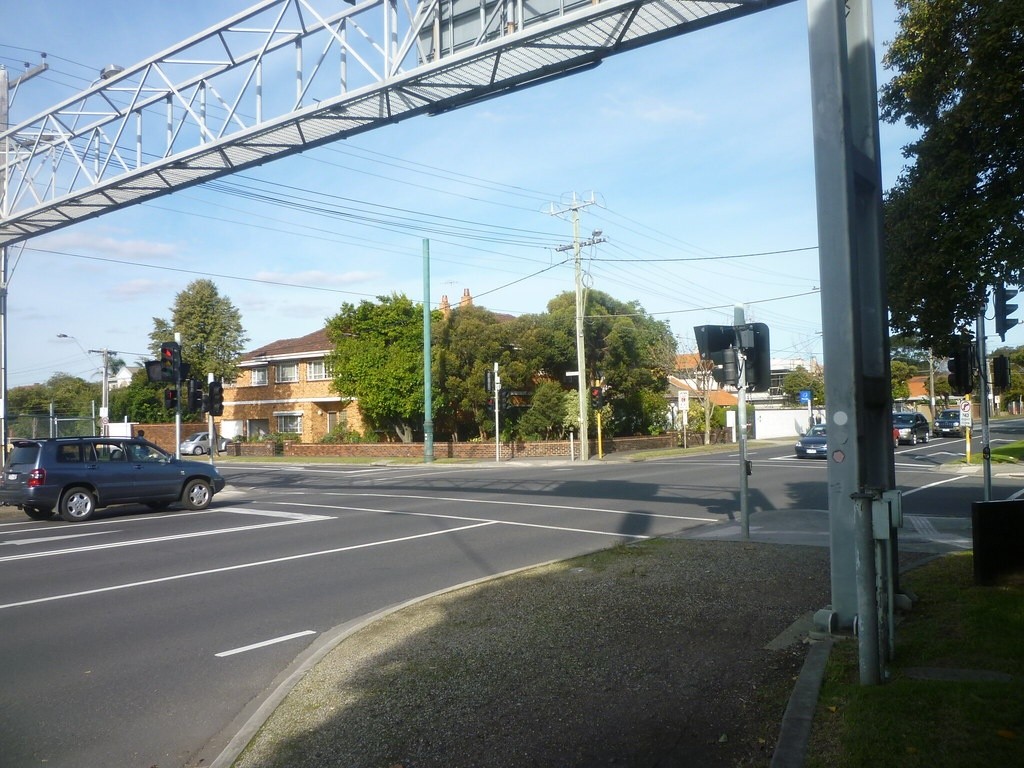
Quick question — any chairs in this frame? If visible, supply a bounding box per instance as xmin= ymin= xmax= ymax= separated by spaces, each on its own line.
xmin=89 ymin=449 xmax=100 ymax=461
xmin=110 ymin=450 xmax=123 ymax=461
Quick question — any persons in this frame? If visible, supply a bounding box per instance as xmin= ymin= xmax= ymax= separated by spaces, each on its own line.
xmin=133 ymin=430 xmax=150 ymax=459
xmin=820 ymin=429 xmax=825 ymax=436
xmin=809 ymin=414 xmax=814 ymax=427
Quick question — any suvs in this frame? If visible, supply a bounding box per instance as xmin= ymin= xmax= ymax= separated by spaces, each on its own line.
xmin=932 ymin=410 xmax=974 ymax=438
xmin=892 ymin=411 xmax=930 ymax=446
xmin=0 ymin=434 xmax=226 ymax=523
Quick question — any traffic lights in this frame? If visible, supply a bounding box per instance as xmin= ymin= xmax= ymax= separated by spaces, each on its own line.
xmin=201 ymin=394 xmax=211 ymax=414
xmin=710 ymin=347 xmax=739 ymax=387
xmin=590 ymin=386 xmax=603 ymax=410
xmin=693 ymin=324 xmax=736 ymax=360
xmin=498 ymin=389 xmax=509 ymax=411
xmin=143 ymin=359 xmax=164 ymax=383
xmin=209 ymin=381 xmax=225 ymax=416
xmin=161 ymin=341 xmax=180 ymax=381
xmin=948 ymin=333 xmax=975 ymax=398
xmin=164 ymin=387 xmax=178 ymax=410
xmin=178 ymin=363 xmax=191 ymax=384
xmin=991 ymin=276 xmax=1019 ymax=343
xmin=187 ymin=379 xmax=203 ymax=413
xmin=487 ymin=397 xmax=496 ymax=412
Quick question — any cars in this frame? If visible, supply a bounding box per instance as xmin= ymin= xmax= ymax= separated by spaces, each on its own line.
xmin=893 ymin=425 xmax=901 ymax=448
xmin=794 ymin=423 xmax=828 ymax=459
xmin=180 ymin=431 xmax=233 ymax=455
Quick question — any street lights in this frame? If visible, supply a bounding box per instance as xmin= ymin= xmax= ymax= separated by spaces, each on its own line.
xmin=57 ymin=332 xmax=111 ymax=457
xmin=573 ymin=228 xmax=605 ymax=461
xmin=0 ymin=63 xmax=126 ymax=476
